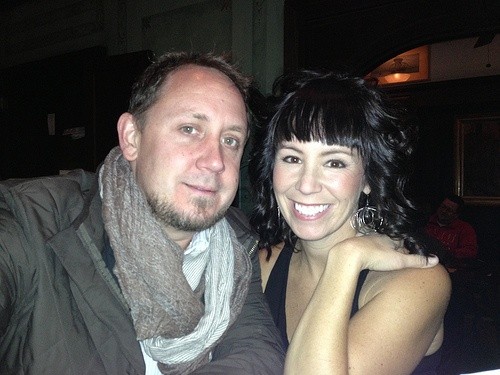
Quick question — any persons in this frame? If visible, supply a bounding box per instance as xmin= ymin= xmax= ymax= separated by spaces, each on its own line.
xmin=397 ymin=195 xmax=480 ymax=276
xmin=0 ymin=51 xmax=290 ymax=375
xmin=389 ymin=59 xmax=407 ymax=72
xmin=248 ymin=69 xmax=500 ymax=375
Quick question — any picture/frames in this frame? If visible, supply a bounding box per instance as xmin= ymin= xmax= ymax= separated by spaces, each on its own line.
xmin=452 ymin=111 xmax=500 ymax=207
